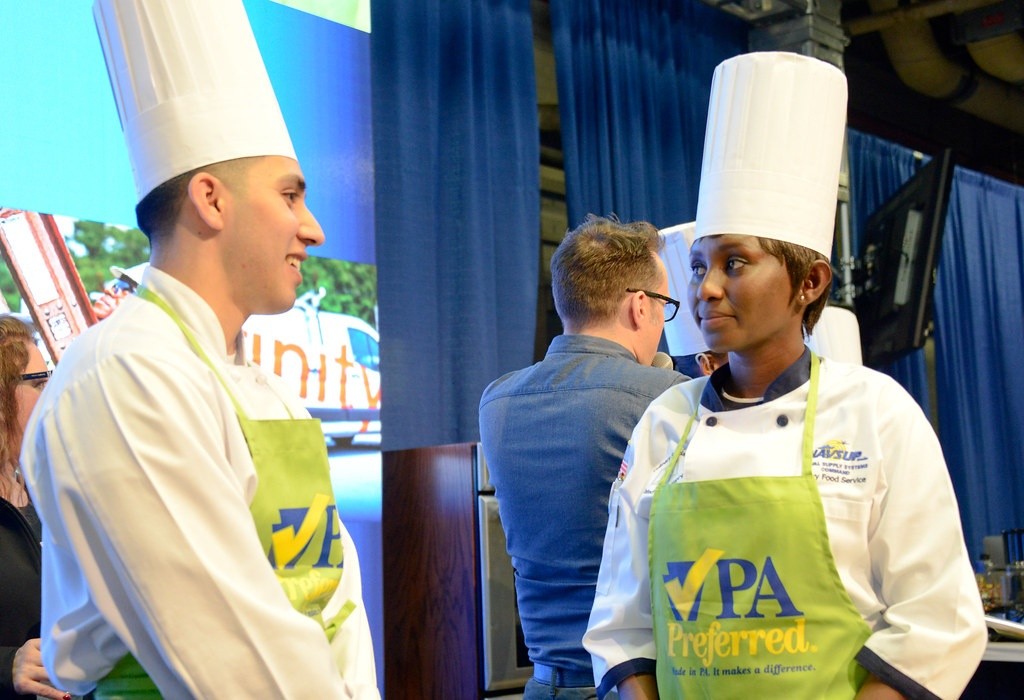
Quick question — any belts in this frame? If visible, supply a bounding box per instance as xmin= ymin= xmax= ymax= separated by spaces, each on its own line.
xmin=533 ymin=662 xmax=595 ymax=687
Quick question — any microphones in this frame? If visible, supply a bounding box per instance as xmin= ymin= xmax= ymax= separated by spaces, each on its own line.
xmin=651 ymin=351 xmax=674 ymax=371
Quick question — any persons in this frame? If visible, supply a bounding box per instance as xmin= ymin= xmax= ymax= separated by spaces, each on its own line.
xmin=477 ymin=217 xmax=695 ymax=699
xmin=0 ymin=313 xmax=71 ymax=700
xmin=580 ymin=200 xmax=989 ymax=699
xmin=21 ymin=120 xmax=377 ymax=700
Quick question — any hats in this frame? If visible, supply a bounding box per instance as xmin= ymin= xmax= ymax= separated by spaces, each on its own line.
xmin=87 ymin=0 xmax=300 ymax=206
xmin=693 ymin=50 xmax=849 ymax=264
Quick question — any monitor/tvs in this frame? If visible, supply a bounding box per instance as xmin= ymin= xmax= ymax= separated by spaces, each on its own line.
xmin=864 ymin=147 xmax=954 ymax=359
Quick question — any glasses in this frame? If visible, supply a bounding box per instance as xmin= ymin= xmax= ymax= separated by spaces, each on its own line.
xmin=627 ymin=287 xmax=680 ymax=322
xmin=12 ymin=369 xmax=54 ymax=382
